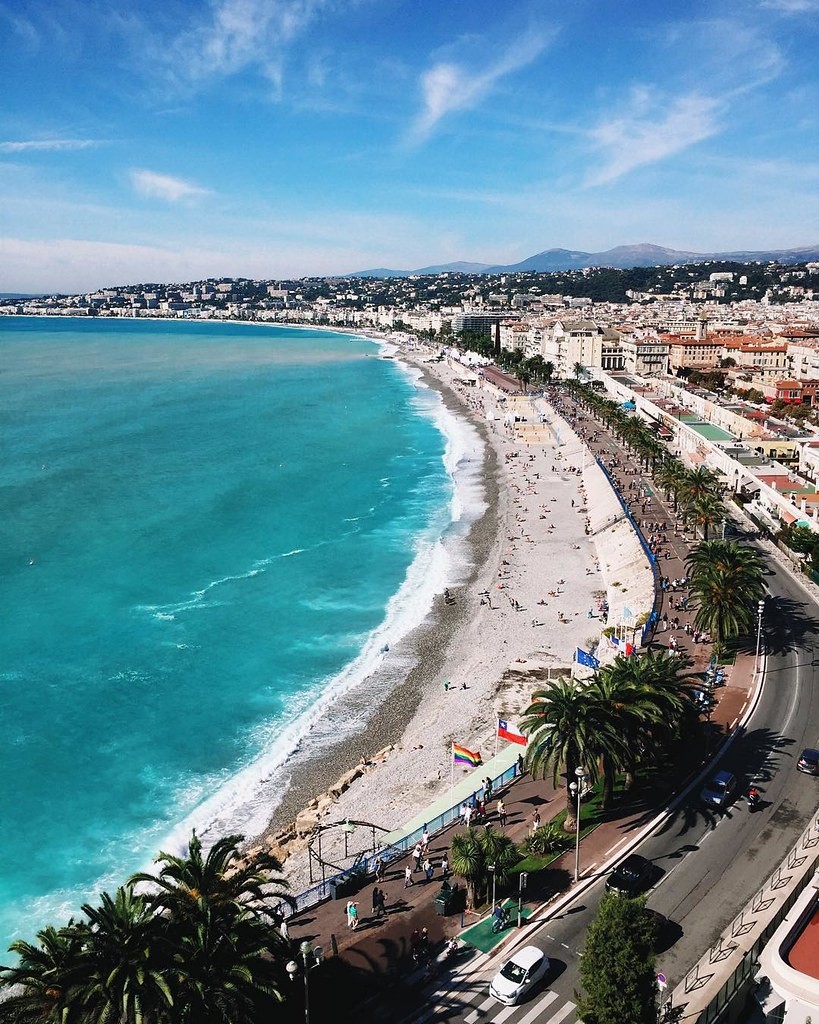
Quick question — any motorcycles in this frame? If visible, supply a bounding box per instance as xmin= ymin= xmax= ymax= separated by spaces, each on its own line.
xmin=748 ymin=794 xmax=759 ymax=812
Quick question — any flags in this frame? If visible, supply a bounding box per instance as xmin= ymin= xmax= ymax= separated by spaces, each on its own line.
xmin=577 ymin=647 xmax=601 ymax=670
xmin=498 ymin=718 xmax=528 ymax=746
xmin=611 ymin=634 xmax=636 ymax=657
xmin=453 ymin=743 xmax=483 ymax=767
xmin=530 ymin=694 xmax=549 ymax=717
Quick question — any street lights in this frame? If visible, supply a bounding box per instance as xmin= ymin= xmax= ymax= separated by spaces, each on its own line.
xmin=721 ymin=515 xmax=727 ymax=541
xmin=286 ymin=941 xmax=323 ymax=1024
xmin=569 ymin=766 xmax=590 ymax=883
xmin=755 ymin=600 xmax=765 ymax=673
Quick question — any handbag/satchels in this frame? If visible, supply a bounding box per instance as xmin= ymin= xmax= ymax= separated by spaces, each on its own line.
xmin=667 ymin=642 xmax=673 ymax=648
xmin=374 ymin=865 xmax=377 ymax=872
xmin=428 ymin=869 xmax=434 ymax=876
xmin=344 ymin=907 xmax=347 ymax=914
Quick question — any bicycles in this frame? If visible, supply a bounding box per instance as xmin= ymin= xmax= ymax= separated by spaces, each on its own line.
xmin=447 ymin=948 xmax=460 ymax=956
xmin=490 ymin=909 xmax=512 ymax=934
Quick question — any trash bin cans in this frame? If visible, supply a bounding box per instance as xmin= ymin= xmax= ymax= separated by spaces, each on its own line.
xmin=432 ymin=881 xmax=461 ymax=915
xmin=328 ymin=875 xmax=351 ymax=900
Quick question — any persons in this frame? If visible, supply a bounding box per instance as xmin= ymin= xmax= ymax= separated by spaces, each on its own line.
xmin=275 ymin=387 xmax=717 ymax=944
xmin=749 ymin=789 xmax=758 ymax=799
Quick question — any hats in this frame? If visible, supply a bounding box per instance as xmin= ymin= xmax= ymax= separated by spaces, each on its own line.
xmin=347 ymin=901 xmax=353 ymax=906
xmin=377 ymin=856 xmax=380 ymax=862
xmin=414 ymin=928 xmax=419 ymax=931
xmin=424 ymin=830 xmax=428 ymax=833
xmin=422 ymin=928 xmax=428 ymax=932
xmin=379 ymin=890 xmax=383 ymax=893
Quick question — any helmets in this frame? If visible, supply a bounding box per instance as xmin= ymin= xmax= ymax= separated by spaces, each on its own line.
xmin=751 ymin=788 xmax=756 ymax=791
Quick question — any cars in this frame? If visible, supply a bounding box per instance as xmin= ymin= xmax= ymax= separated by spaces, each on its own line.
xmin=699 ymin=769 xmax=738 ymax=809
xmin=488 ymin=945 xmax=550 ymax=1006
xmin=605 ymin=854 xmax=653 ymax=898
xmin=797 ymin=748 xmax=819 ymax=776
